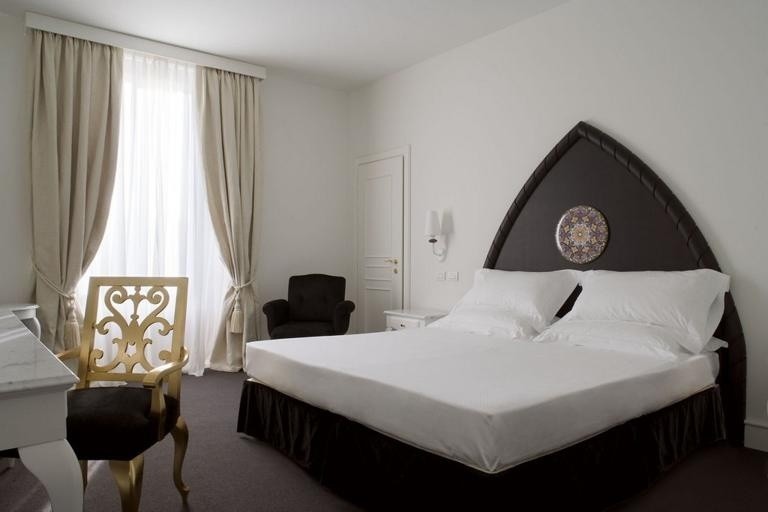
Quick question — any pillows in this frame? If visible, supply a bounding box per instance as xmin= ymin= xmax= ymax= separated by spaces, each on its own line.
xmin=428 ymin=267 xmax=730 ymax=361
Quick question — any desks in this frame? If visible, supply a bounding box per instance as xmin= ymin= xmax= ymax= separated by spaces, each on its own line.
xmin=1 ymin=306 xmax=80 ymax=510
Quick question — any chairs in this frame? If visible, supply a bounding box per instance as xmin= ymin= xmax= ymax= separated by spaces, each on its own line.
xmin=261 ymin=273 xmax=356 ymax=341
xmin=53 ymin=274 xmax=190 ymax=511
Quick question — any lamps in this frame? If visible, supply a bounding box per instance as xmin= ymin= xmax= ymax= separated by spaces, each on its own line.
xmin=423 ymin=210 xmax=448 ymax=258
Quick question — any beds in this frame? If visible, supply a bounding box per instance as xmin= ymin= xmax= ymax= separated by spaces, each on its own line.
xmin=237 ymin=117 xmax=749 ymax=512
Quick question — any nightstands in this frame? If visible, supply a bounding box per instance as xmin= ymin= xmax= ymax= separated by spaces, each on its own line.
xmin=382 ymin=307 xmax=446 ymax=331
